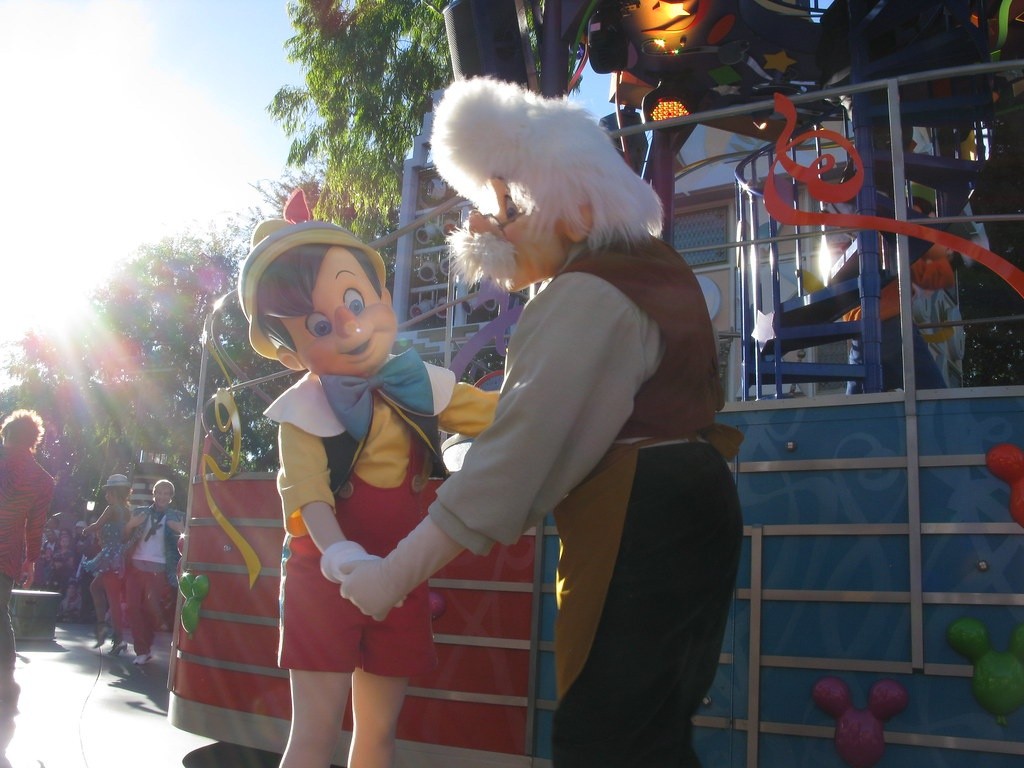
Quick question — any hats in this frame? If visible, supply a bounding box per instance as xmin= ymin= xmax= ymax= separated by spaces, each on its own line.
xmin=75 ymin=521 xmax=87 ymax=529
xmin=101 ymin=474 xmax=131 ymax=490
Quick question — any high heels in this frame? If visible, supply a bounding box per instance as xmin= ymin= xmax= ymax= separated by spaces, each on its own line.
xmin=93 ymin=627 xmax=111 ymax=649
xmin=109 ymin=640 xmax=128 ymax=656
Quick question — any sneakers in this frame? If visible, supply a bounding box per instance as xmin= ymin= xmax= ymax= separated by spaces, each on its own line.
xmin=132 ymin=652 xmax=151 ymax=666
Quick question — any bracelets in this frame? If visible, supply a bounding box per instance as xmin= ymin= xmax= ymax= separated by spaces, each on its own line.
xmin=83 ymin=529 xmax=86 ymax=535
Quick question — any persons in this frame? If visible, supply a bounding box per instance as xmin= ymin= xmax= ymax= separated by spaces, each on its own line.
xmin=338 ymin=79 xmax=726 ymax=768
xmin=81 ymin=474 xmax=131 ymax=655
xmin=105 ymin=590 xmax=127 ymax=628
xmin=841 ymin=195 xmax=990 ymax=396
xmin=125 ymin=479 xmax=185 ymax=666
xmin=0 ymin=412 xmax=55 ymax=672
xmin=33 ymin=513 xmax=97 ymax=623
xmin=238 ymin=216 xmax=501 ymax=768
